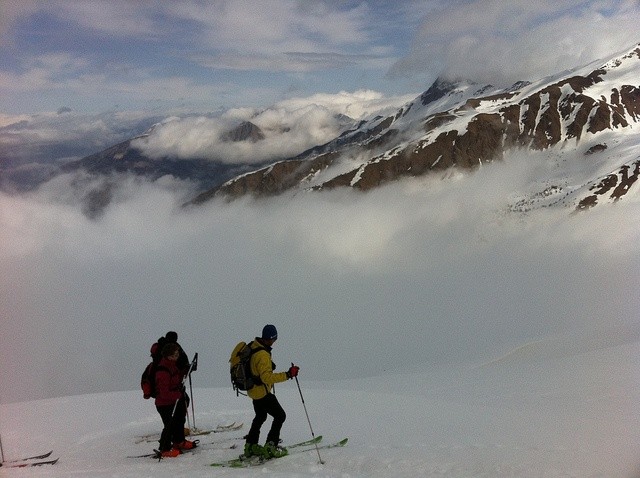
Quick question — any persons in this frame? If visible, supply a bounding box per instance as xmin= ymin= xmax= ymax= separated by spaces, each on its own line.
xmin=151 ymin=331 xmax=190 ymax=436
xmin=244 ymin=323 xmax=299 ymax=459
xmin=155 ymin=343 xmax=196 ymax=456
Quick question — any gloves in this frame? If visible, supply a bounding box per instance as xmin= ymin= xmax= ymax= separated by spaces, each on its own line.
xmin=286 ymin=366 xmax=300 ymax=380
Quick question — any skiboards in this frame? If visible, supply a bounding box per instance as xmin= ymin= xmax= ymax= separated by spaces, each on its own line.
xmin=206 ymin=431 xmax=348 ymax=469
xmin=132 ymin=422 xmax=244 ymax=444
xmin=0 ymin=440 xmax=60 ymax=468
xmin=127 ymin=434 xmax=244 ymax=465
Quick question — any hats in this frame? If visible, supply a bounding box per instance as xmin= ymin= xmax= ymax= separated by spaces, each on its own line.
xmin=262 ymin=323 xmax=277 ymax=338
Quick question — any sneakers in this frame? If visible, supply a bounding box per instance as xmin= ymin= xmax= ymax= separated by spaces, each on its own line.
xmin=264 ymin=442 xmax=287 ymax=457
xmin=244 ymin=442 xmax=264 ymax=456
xmin=160 ymin=447 xmax=179 ymax=456
xmin=173 ymin=438 xmax=192 ymax=449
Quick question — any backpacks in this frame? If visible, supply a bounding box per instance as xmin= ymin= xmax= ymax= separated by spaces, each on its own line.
xmin=228 ymin=340 xmax=271 ymax=395
xmin=141 ymin=362 xmax=172 ymax=400
